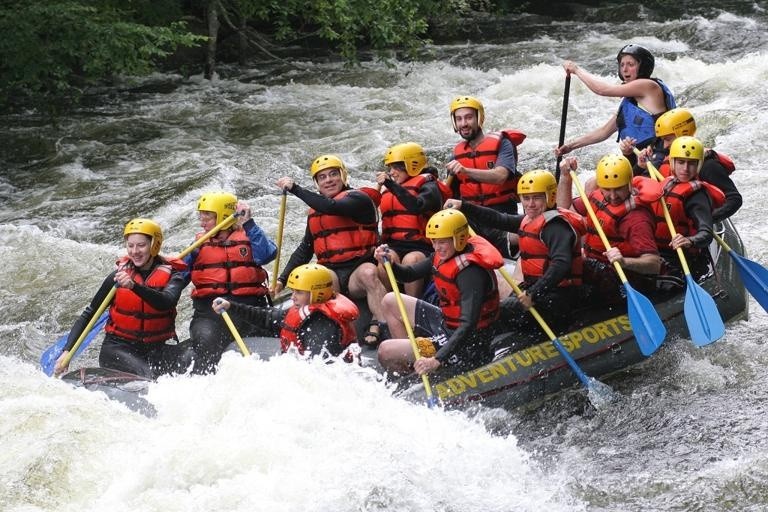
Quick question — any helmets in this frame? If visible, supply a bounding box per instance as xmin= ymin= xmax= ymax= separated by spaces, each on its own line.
xmin=195 ymin=191 xmax=240 ymax=230
xmin=655 ymin=108 xmax=697 ymax=138
xmin=124 ymin=218 xmax=163 ymax=257
xmin=286 ymin=264 xmax=334 ymax=304
xmin=518 ymin=170 xmax=558 ymax=209
xmin=451 ymin=97 xmax=485 ymax=132
xmin=311 ymin=155 xmax=348 ymax=192
xmin=426 ymin=208 xmax=469 ymax=251
xmin=596 ymin=153 xmax=633 ymax=194
xmin=385 ymin=141 xmax=429 ymax=177
xmin=617 ymin=44 xmax=655 ymax=81
xmin=669 ymin=136 xmax=704 ymax=174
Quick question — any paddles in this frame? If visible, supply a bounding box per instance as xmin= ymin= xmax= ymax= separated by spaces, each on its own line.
xmin=628 ymin=142 xmax=768 ymax=318
xmin=39 ymin=205 xmax=246 ymax=379
xmin=380 ymin=250 xmax=441 ymax=409
xmin=565 ymin=165 xmax=669 ymax=359
xmin=446 ymin=201 xmax=624 ymax=414
xmin=641 ymin=153 xmax=728 ymax=349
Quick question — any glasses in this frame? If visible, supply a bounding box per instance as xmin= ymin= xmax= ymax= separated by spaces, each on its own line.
xmin=317 ymin=171 xmax=339 ymax=181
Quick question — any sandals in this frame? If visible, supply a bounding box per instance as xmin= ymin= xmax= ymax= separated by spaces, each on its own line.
xmin=364 ymin=320 xmax=384 ymax=346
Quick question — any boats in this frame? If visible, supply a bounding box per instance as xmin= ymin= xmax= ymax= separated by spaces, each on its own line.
xmin=62 ymin=217 xmax=748 ymax=424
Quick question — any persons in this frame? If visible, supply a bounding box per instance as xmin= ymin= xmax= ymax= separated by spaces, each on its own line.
xmin=556 ymin=151 xmax=661 ymax=310
xmin=440 ymin=92 xmax=522 ymax=216
xmin=266 ymin=156 xmax=389 ymax=345
xmin=206 ymin=262 xmax=362 ymax=363
xmin=646 ymin=131 xmax=713 ymax=294
xmin=442 ymin=164 xmax=587 ymax=321
xmin=631 ymin=105 xmax=745 ymax=224
xmin=372 ymin=209 xmax=503 ymax=378
xmin=374 ymin=140 xmax=444 ymax=299
xmin=173 ymin=192 xmax=280 ymax=348
xmin=50 ymin=212 xmax=196 ymax=381
xmin=557 ymin=43 xmax=678 ymax=174
